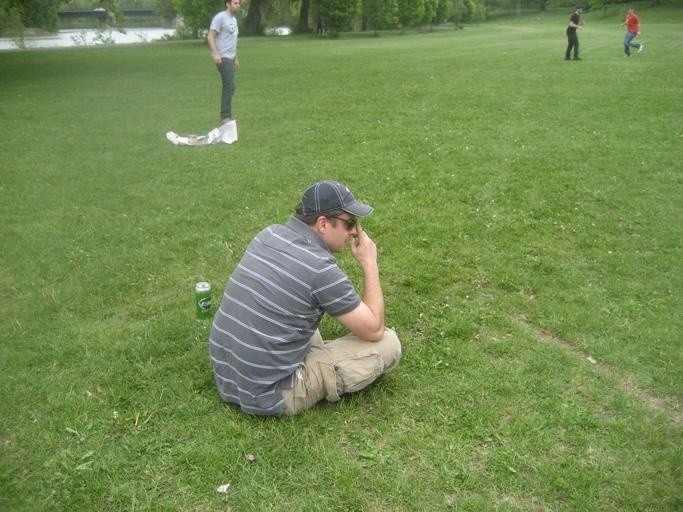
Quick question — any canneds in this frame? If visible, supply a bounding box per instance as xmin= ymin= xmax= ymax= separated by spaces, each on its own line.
xmin=195 ymin=282 xmax=212 ymax=320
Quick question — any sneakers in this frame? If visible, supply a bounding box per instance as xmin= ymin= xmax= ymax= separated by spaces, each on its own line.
xmin=637 ymin=44 xmax=643 ymax=53
xmin=565 ymin=56 xmax=582 ymax=60
xmin=221 ymin=117 xmax=232 ymax=124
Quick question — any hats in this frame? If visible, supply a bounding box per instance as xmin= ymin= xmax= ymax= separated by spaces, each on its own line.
xmin=301 ymin=180 xmax=375 ymax=218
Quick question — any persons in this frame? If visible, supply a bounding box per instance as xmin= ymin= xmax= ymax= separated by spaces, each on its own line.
xmin=619 ymin=6 xmax=644 ymax=59
xmin=563 ymin=3 xmax=586 ymax=61
xmin=207 ymin=180 xmax=402 ymax=419
xmin=206 ymin=0 xmax=242 ymax=126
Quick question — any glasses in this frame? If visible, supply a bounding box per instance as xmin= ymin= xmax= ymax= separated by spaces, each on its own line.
xmin=333 ymin=215 xmax=357 ymax=231
